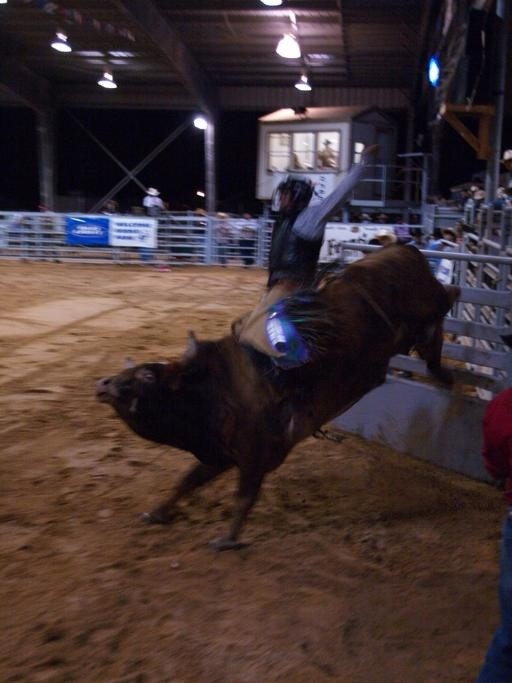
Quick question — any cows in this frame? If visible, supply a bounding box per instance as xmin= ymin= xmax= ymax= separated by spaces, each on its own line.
xmin=94 ymin=235 xmax=461 ymax=551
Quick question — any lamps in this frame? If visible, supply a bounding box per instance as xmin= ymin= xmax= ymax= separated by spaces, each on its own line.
xmin=49 ymin=26 xmax=118 ymax=90
xmin=277 ymin=32 xmax=313 ymax=93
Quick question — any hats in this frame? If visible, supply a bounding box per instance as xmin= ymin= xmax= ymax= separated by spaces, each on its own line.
xmin=322 ymin=139 xmax=332 ymax=145
xmin=375 ymin=229 xmax=398 ymax=243
xmin=145 ymin=187 xmax=161 ymax=196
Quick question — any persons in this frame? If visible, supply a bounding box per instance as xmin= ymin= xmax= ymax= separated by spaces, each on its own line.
xmin=424 ymin=184 xmax=512 ymax=318
xmin=472 ymin=330 xmax=512 ymax=683
xmin=318 ymin=138 xmax=339 ymax=169
xmin=231 ymin=142 xmax=381 ymax=378
xmin=496 ymin=148 xmax=512 ymax=172
xmin=348 ymin=211 xmax=424 ymax=249
xmin=101 ymin=186 xmax=257 ymax=267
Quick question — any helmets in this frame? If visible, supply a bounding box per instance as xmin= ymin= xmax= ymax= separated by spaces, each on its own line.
xmin=267 ymin=178 xmax=314 ymax=219
